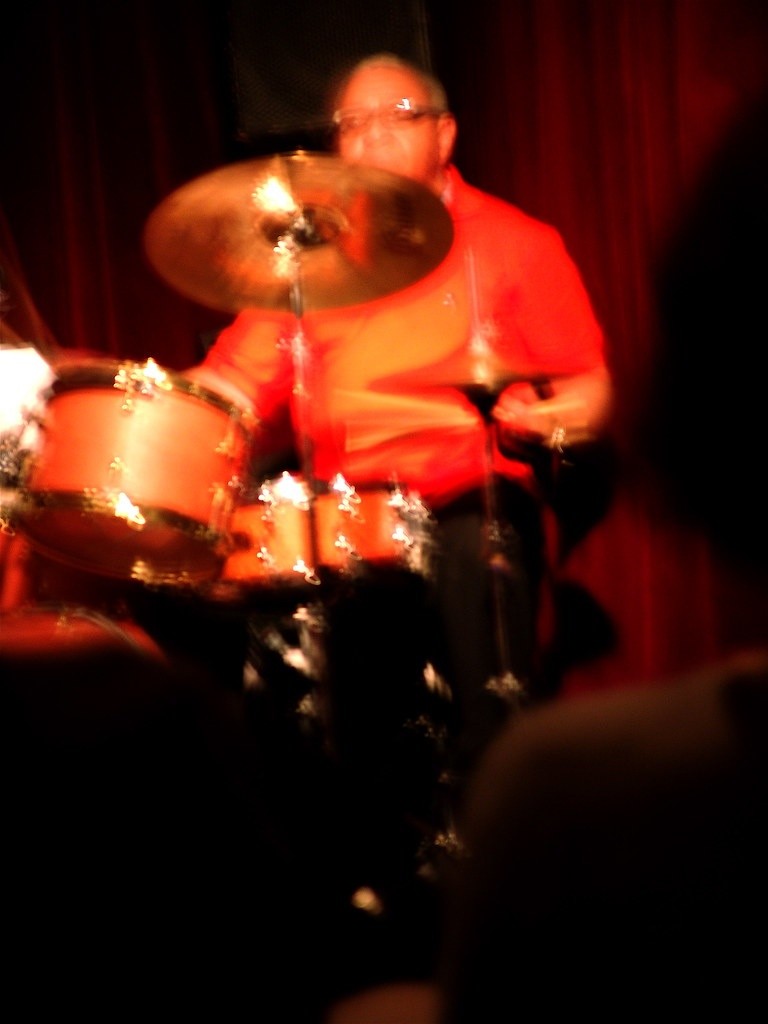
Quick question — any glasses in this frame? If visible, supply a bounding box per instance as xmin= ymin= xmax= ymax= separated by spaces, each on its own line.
xmin=333 ymin=102 xmax=441 ymax=137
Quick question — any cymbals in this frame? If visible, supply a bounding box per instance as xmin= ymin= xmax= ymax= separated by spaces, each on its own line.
xmin=141 ymin=154 xmax=456 ymax=316
xmin=363 ymin=338 xmax=596 ymax=394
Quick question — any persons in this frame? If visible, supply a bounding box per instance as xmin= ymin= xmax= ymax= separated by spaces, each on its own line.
xmin=189 ymin=57 xmax=614 ymax=644
xmin=431 ymin=96 xmax=768 ymax=1024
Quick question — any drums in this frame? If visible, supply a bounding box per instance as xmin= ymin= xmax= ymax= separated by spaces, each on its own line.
xmin=5 ymin=361 xmax=261 ymax=588
xmin=220 ymin=469 xmax=433 ymax=588
